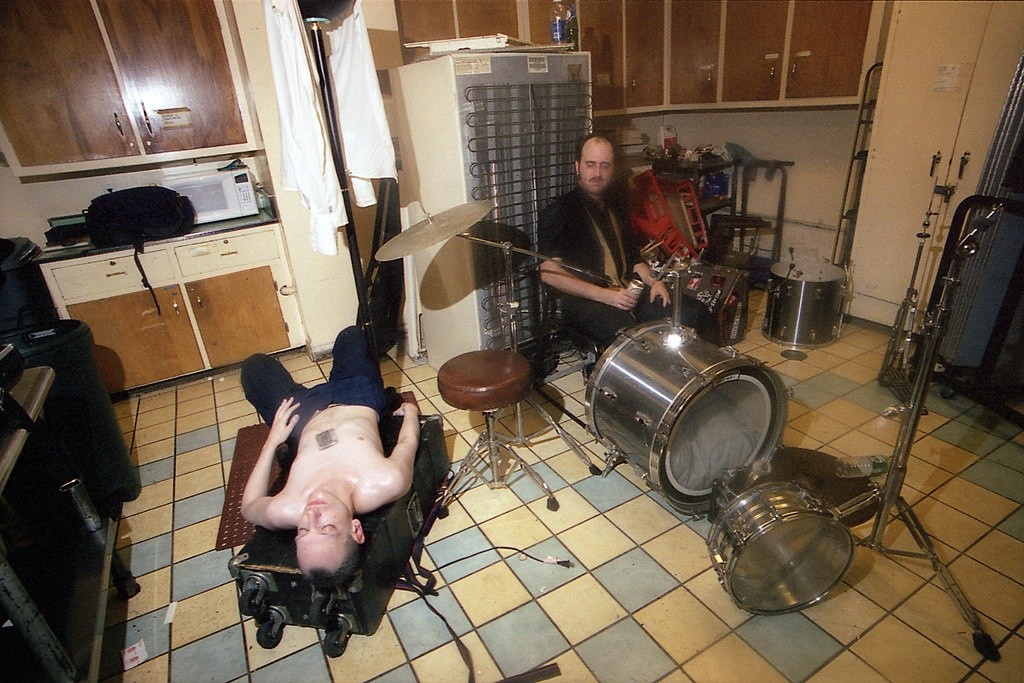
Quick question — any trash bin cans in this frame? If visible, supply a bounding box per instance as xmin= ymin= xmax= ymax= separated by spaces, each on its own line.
xmin=0 ymin=236 xmax=59 ymax=328
xmin=1 ymin=319 xmax=140 ymax=505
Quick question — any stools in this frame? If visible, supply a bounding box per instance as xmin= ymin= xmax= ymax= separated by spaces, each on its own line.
xmin=438 ymin=350 xmax=559 ymax=523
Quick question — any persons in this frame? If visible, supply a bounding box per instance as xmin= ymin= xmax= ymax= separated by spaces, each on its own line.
xmin=239 ymin=326 xmax=423 ymax=595
xmin=535 ymin=134 xmax=673 ymax=382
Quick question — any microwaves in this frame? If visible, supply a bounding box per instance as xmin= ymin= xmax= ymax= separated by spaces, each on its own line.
xmin=161 ymin=165 xmax=261 ymax=225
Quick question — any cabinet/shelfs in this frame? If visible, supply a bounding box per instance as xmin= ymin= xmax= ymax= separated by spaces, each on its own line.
xmin=520 ymin=0 xmax=580 ymax=54
xmin=719 ymin=0 xmax=892 ymax=109
xmin=0 ymin=365 xmax=142 ymax=683
xmin=664 ymin=0 xmax=723 ymax=112
xmin=391 ymin=1 xmax=518 ymax=79
xmin=575 ymin=0 xmax=662 ymax=113
xmin=656 ymin=154 xmax=735 ymax=248
xmin=0 ymin=1 xmax=260 ymax=182
xmin=27 ymin=209 xmax=310 ymax=404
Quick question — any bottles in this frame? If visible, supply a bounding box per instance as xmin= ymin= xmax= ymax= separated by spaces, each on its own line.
xmin=550 ymin=0 xmax=576 ymax=49
xmin=657 ymin=125 xmax=676 ymax=152
